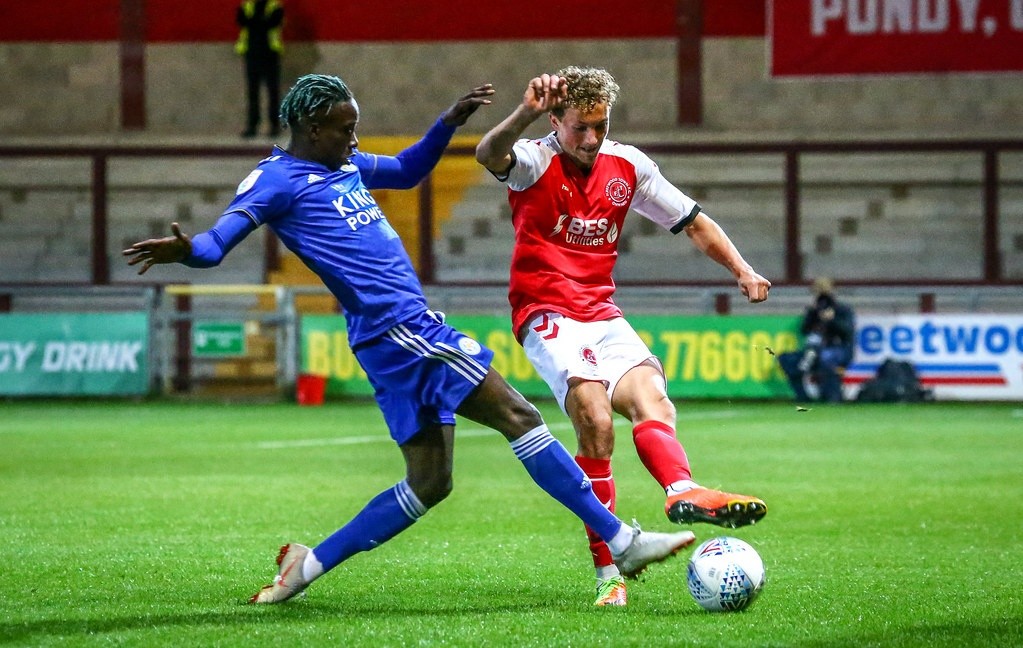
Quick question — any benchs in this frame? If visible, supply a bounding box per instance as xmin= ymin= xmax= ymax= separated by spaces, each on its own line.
xmin=0 ymin=138 xmax=1023 ymax=313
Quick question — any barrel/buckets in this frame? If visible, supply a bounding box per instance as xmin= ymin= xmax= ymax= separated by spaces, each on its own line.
xmin=297 ymin=374 xmax=325 ymax=404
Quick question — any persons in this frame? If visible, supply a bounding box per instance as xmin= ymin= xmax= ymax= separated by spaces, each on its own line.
xmin=120 ymin=75 xmax=696 ymax=605
xmin=475 ymin=67 xmax=772 ymax=605
xmin=778 ymin=276 xmax=856 ymax=401
xmin=232 ymin=0 xmax=288 ymax=140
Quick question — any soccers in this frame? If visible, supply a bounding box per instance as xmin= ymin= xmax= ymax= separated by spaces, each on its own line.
xmin=687 ymin=537 xmax=766 ymax=611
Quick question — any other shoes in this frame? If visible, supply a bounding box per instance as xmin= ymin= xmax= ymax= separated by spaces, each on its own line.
xmin=241 ymin=126 xmax=257 ymax=138
xmin=268 ymin=124 xmax=282 ymax=138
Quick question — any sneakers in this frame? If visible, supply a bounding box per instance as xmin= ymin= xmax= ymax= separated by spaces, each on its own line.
xmin=250 ymin=544 xmax=308 ymax=603
xmin=593 ymin=576 xmax=627 ymax=606
xmin=665 ymin=488 xmax=767 ymax=529
xmin=614 ymin=518 xmax=695 ymax=579
